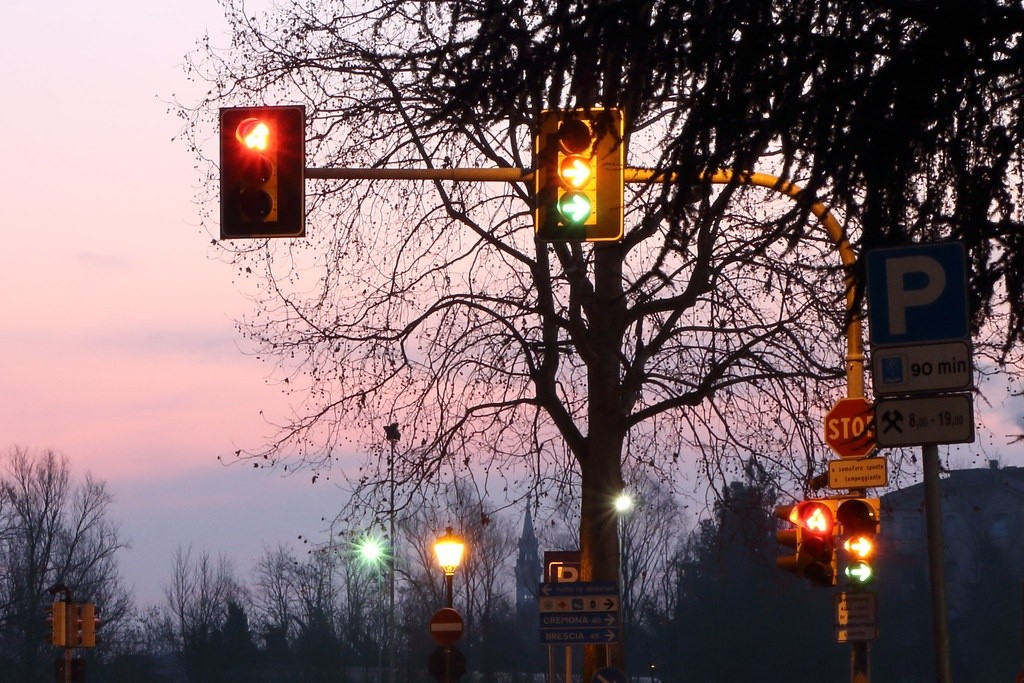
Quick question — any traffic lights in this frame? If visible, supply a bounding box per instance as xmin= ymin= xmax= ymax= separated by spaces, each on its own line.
xmin=837 ymin=497 xmax=879 ymax=587
xmin=67 ymin=603 xmax=82 ymax=648
xmin=775 ymin=503 xmax=801 ymax=578
xmin=534 ymin=107 xmax=623 ymax=243
xmin=80 ymin=602 xmax=102 ymax=647
xmin=218 ymin=104 xmax=307 ymax=240
xmin=797 ymin=501 xmax=837 ymax=587
xmin=44 ymin=602 xmax=66 ymax=646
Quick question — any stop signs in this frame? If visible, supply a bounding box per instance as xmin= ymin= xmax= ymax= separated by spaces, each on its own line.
xmin=428 ymin=608 xmax=463 ymax=647
xmin=824 ymin=397 xmax=877 ymax=458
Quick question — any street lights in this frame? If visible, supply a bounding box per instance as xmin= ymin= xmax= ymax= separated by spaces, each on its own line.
xmin=382 ymin=426 xmax=401 ymax=683
xmin=433 ymin=525 xmax=464 ymax=683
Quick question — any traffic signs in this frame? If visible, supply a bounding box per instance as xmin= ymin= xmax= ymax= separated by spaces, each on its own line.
xmin=539 ymin=594 xmax=619 ymax=613
xmin=538 ymin=612 xmax=621 ymax=630
xmin=538 ymin=582 xmax=619 ymax=597
xmin=539 ymin=628 xmax=621 ymax=645
